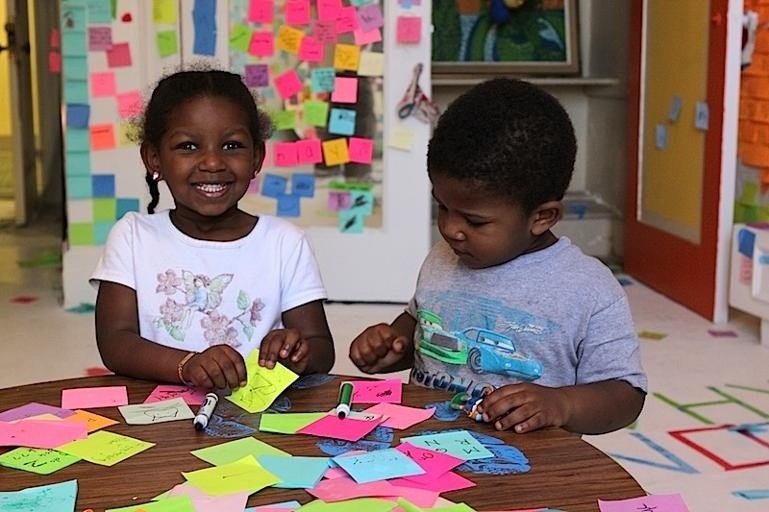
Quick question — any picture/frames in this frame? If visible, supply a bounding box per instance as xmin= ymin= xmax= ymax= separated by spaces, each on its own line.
xmin=428 ymin=0 xmax=586 ymax=76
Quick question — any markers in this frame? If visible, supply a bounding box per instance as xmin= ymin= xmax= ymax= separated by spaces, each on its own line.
xmin=336 ymin=381 xmax=355 ymax=419
xmin=193 ymin=391 xmax=219 ymax=432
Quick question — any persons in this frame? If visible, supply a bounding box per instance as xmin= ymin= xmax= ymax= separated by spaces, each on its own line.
xmin=89 ymin=72 xmax=334 ymax=392
xmin=179 ymin=274 xmax=211 ymax=328
xmin=350 ymin=79 xmax=647 ymax=433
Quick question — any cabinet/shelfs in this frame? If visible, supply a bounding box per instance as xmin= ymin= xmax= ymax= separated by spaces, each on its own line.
xmin=427 ymin=71 xmax=626 ymax=225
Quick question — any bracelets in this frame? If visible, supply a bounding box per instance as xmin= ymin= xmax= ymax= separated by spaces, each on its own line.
xmin=178 ymin=352 xmax=198 ymax=388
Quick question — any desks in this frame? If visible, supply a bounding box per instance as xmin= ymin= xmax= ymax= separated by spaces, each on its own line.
xmin=0 ymin=373 xmax=654 ymax=510
